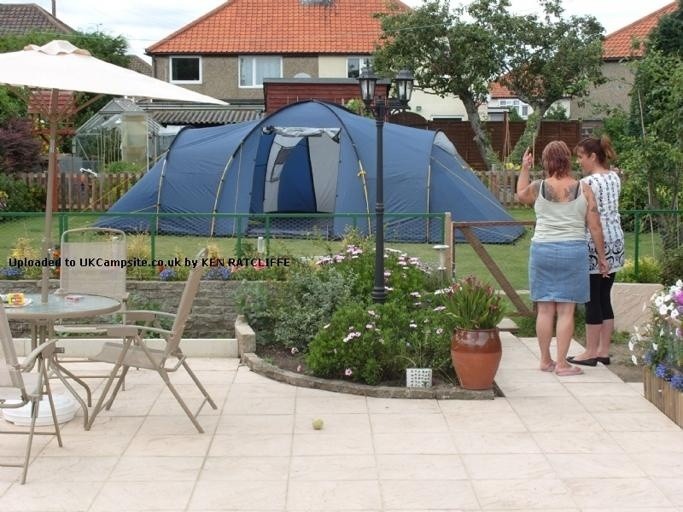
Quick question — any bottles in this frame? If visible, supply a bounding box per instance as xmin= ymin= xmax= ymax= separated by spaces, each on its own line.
xmin=258 ymin=233 xmax=264 ymax=253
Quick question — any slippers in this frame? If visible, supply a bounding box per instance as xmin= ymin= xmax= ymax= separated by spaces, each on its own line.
xmin=556 ymin=365 xmax=583 ymax=375
xmin=541 ymin=360 xmax=556 ymax=372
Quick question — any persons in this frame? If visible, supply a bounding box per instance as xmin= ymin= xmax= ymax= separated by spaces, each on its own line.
xmin=565 ymin=138 xmax=625 ymax=368
xmin=517 ymin=141 xmax=609 ymax=373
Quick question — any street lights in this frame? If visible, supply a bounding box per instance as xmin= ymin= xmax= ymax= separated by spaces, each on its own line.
xmin=355 ymin=61 xmax=417 ymax=308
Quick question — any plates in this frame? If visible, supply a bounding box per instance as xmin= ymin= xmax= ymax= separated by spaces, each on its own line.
xmin=4 ymin=298 xmax=32 ymax=308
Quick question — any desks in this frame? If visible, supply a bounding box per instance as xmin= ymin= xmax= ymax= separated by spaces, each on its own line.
xmin=1 ymin=294 xmax=122 ymax=426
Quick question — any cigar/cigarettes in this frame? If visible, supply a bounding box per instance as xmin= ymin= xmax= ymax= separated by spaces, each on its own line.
xmin=605 ymin=275 xmax=610 ymax=278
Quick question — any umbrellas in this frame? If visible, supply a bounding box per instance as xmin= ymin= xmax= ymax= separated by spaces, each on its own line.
xmin=0 ymin=38 xmax=231 ymax=304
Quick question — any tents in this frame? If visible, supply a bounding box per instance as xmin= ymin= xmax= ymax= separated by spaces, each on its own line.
xmin=82 ymin=95 xmax=528 ymax=244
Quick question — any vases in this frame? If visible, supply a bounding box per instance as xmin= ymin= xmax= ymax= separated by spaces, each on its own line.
xmin=405 ymin=367 xmax=433 ymax=389
xmin=643 ymin=364 xmax=683 ymax=430
xmin=450 ymin=326 xmax=502 ymax=390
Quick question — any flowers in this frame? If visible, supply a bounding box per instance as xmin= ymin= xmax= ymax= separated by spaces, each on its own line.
xmin=394 ymin=318 xmax=451 ymax=369
xmin=439 ymin=274 xmax=510 ymax=329
xmin=628 ymin=277 xmax=682 ymax=392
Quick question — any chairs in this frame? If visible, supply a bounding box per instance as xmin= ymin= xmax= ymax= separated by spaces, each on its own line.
xmin=0 ymin=295 xmax=62 ymax=485
xmin=48 ymin=227 xmax=139 ymax=391
xmin=87 ymin=249 xmax=217 ymax=434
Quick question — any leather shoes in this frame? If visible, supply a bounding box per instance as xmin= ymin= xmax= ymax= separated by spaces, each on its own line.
xmin=597 ymin=358 xmax=610 ymax=364
xmin=567 ymin=356 xmax=596 ymax=366
